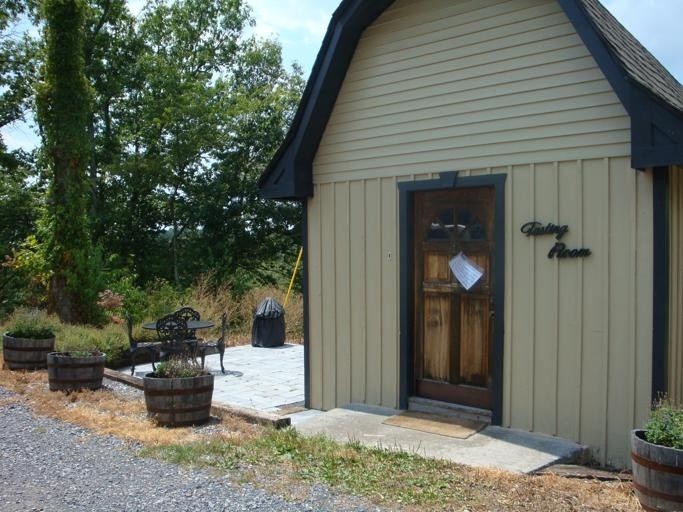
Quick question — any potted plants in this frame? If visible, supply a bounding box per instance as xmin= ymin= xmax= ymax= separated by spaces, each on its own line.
xmin=3 ymin=308 xmax=107 ymax=392
xmin=144 ymin=354 xmax=214 ymax=424
xmin=631 ymin=391 xmax=683 ymax=510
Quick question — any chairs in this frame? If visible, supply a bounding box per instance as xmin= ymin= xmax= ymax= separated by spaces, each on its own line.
xmin=125 ymin=307 xmax=227 ymax=376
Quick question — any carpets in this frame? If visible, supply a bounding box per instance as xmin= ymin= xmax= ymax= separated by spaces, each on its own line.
xmin=382 ymin=407 xmax=489 ymax=441
